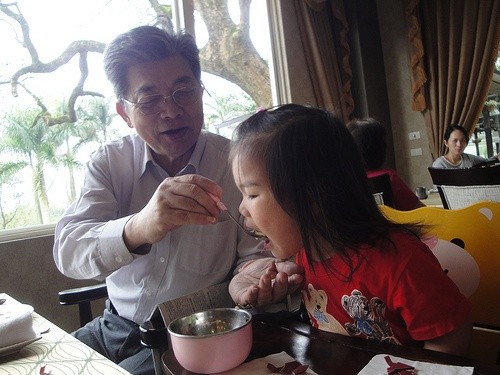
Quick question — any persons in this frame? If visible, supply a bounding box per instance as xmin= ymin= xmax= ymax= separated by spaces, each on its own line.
xmin=228 ymin=103 xmax=500 ymax=358
xmin=52 ymin=27 xmax=305 ymax=375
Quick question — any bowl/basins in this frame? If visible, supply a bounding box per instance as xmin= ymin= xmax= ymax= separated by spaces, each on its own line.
xmin=167 ymin=308 xmax=252 ymax=373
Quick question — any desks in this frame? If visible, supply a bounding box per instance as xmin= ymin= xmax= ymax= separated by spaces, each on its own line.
xmin=158 ymin=303 xmax=500 ymax=375
xmin=0 ymin=310 xmax=134 ymax=375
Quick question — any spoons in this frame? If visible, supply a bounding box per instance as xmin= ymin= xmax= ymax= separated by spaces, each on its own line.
xmin=207 ymin=192 xmax=268 ymax=240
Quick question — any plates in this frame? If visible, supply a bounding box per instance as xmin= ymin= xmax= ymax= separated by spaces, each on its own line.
xmin=0 ymin=336 xmax=43 ymax=356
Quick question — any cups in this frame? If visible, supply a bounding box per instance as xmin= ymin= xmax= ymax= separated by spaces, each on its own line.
xmin=416 ymin=187 xmax=426 ymax=198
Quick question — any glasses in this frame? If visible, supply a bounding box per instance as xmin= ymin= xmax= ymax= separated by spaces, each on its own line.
xmin=121 ymin=79 xmax=205 ymax=115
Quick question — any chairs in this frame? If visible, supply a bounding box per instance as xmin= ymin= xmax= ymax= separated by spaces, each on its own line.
xmin=427 ymin=164 xmax=500 ymax=211
xmin=56 ymin=282 xmax=172 ymax=375
